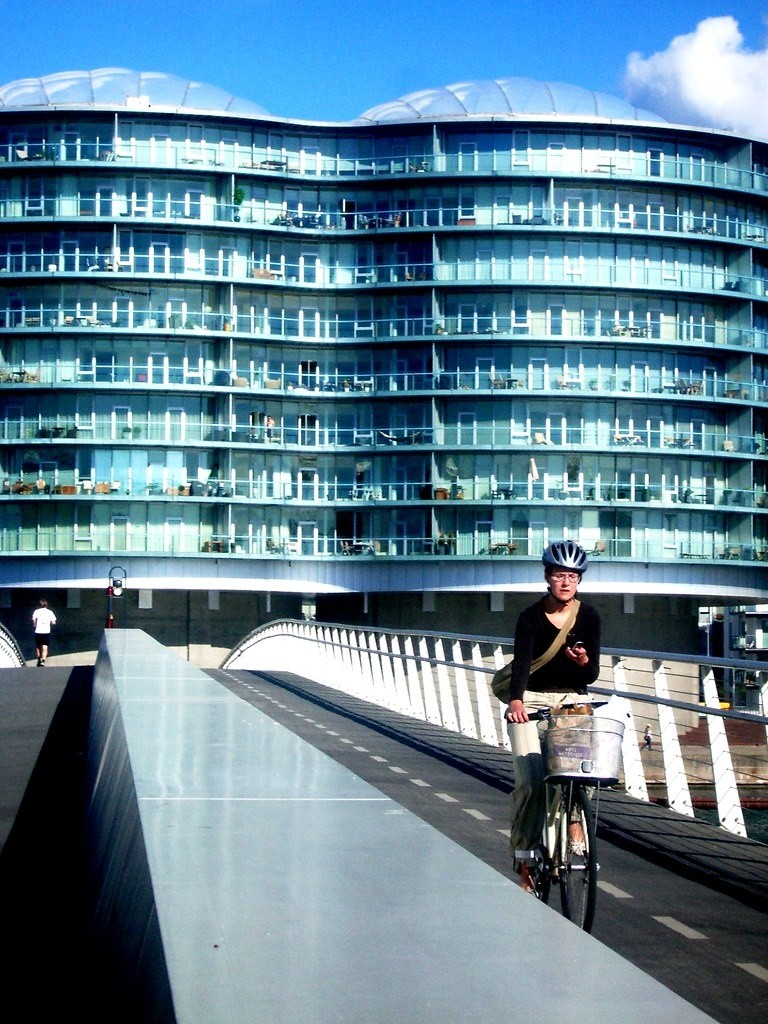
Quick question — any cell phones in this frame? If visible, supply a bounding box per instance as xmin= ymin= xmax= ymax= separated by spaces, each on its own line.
xmin=571 ymin=641 xmax=583 ymax=651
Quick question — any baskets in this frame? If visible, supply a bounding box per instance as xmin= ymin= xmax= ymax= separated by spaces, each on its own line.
xmin=537 ymin=714 xmax=625 ymax=788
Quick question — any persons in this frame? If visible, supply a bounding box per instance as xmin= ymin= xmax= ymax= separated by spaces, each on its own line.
xmin=267 ymin=415 xmax=275 ymax=444
xmin=640 ymin=724 xmax=653 ymax=751
xmin=31 ymin=599 xmax=57 ymax=667
xmin=504 ymin=540 xmax=602 ymax=899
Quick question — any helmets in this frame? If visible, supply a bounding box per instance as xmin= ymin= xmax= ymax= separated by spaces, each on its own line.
xmin=542 ymin=540 xmax=588 ymax=572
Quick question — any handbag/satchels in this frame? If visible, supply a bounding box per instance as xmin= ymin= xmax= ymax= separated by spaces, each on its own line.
xmin=545 ymin=705 xmax=596 ymax=771
xmin=491 ymin=660 xmax=512 ymax=705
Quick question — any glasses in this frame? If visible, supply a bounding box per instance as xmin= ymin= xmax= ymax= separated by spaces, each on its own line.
xmin=550 ymin=574 xmax=580 ymax=583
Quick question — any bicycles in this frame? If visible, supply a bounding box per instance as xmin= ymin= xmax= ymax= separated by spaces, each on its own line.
xmin=507 ymin=699 xmax=626 ymax=936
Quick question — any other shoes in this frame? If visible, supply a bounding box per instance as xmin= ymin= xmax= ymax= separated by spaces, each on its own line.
xmin=519 ymin=871 xmax=542 ymax=901
xmin=38 ymin=659 xmax=45 ymax=666
xmin=571 ymin=822 xmax=587 ymax=857
